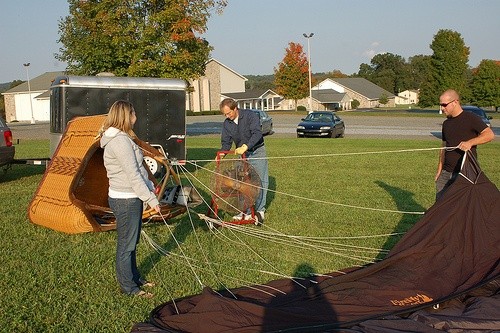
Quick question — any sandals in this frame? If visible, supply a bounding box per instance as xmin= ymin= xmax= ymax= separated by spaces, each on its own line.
xmin=140 ymin=281 xmax=156 ymax=288
xmin=133 ymin=291 xmax=154 ymax=299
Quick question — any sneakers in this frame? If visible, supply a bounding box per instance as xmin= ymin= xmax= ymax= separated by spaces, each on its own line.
xmin=232 ymin=210 xmax=264 ymax=221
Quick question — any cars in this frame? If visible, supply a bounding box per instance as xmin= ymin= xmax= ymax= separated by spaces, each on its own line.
xmin=0 ymin=116 xmax=16 ymax=169
xmin=296 ymin=111 xmax=346 ymax=139
xmin=461 ymin=105 xmax=494 ymax=130
xmin=246 ymin=109 xmax=274 ymax=137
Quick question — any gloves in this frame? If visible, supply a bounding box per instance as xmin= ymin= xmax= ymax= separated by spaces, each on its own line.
xmin=234 ymin=144 xmax=248 ymax=155
xmin=215 ymin=154 xmax=225 ymax=160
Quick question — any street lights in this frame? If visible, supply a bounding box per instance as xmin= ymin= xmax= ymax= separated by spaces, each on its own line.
xmin=303 ymin=32 xmax=315 ymax=114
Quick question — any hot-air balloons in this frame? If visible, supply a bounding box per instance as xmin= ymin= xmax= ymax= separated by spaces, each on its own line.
xmin=28 ymin=113 xmax=500 ymax=333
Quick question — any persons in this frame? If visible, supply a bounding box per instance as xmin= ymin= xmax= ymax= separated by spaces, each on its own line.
xmin=98 ymin=100 xmax=160 ymax=298
xmin=435 ymin=88 xmax=494 ymax=202
xmin=215 ymin=98 xmax=268 ymax=220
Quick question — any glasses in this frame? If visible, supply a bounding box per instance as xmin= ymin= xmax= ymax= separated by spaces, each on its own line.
xmin=439 ymin=100 xmax=459 ymax=107
